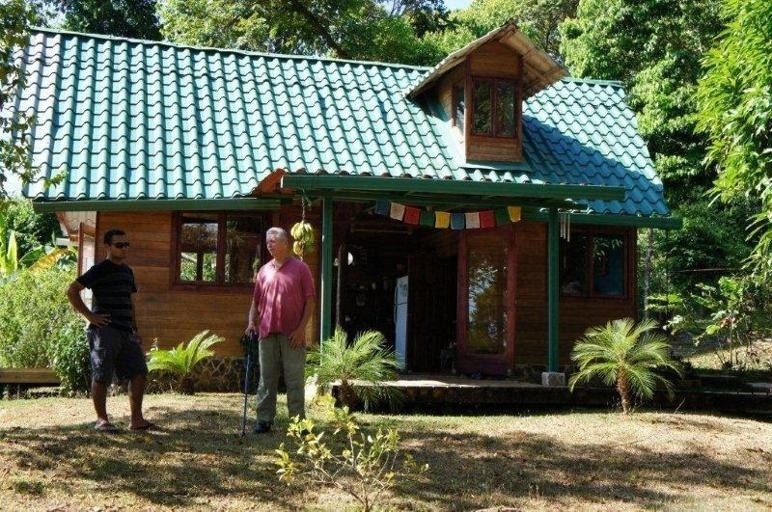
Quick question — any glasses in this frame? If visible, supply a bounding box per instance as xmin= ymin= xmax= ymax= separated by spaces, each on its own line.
xmin=107 ymin=241 xmax=132 ymax=250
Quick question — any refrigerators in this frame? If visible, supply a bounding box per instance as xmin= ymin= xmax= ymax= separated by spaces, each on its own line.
xmin=394 ymin=276 xmax=408 ymax=367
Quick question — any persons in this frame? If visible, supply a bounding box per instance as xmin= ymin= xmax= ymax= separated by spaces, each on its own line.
xmin=64 ymin=226 xmax=158 ymax=434
xmin=243 ymin=225 xmax=317 ymax=435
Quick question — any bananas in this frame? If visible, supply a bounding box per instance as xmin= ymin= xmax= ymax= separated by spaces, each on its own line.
xmin=290 ymin=220 xmax=316 ymax=256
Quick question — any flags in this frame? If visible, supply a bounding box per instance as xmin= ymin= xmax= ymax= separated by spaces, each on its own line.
xmin=434 ymin=210 xmax=451 ymax=229
xmin=507 ymin=204 xmax=522 ymax=223
xmin=419 ymin=210 xmax=435 ymax=228
xmin=403 ymin=206 xmax=422 ymax=225
xmin=389 ymin=201 xmax=406 ymax=222
xmin=374 ymin=196 xmax=390 ymax=216
xmin=464 ymin=211 xmax=481 ymax=230
xmin=450 ymin=212 xmax=466 ymax=231
xmin=494 ymin=206 xmax=510 ymax=228
xmin=479 ymin=208 xmax=495 ymax=230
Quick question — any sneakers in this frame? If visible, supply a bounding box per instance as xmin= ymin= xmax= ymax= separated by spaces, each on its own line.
xmin=250 ymin=423 xmax=274 ymax=436
xmin=302 ymin=427 xmax=313 ymax=436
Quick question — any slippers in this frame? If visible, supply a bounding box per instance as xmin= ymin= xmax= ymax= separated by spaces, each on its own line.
xmin=129 ymin=419 xmax=161 ymax=432
xmin=94 ymin=420 xmax=118 ymax=433
